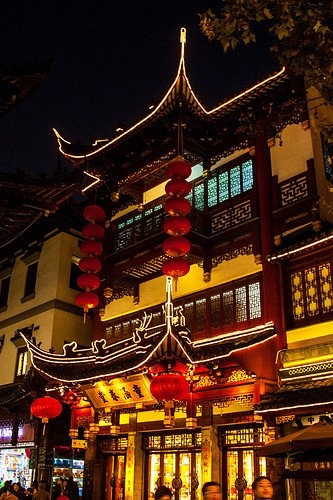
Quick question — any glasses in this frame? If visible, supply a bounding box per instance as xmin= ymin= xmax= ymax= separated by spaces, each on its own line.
xmin=203 ymin=489 xmax=221 ymax=495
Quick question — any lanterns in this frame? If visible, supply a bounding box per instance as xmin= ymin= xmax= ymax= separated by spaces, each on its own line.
xmin=164 ymin=178 xmax=191 ymax=197
xmin=163 ymin=196 xmax=192 ymax=217
xmin=161 ymin=235 xmax=191 ymax=257
xmin=75 ymin=291 xmax=99 ymax=323
xmin=167 ymin=160 xmax=192 ymax=180
xmin=151 ymin=372 xmax=191 ymax=425
xmin=77 ymin=272 xmax=101 ymax=291
xmin=163 ymin=216 xmax=193 ymax=237
xmin=81 ymin=224 xmax=104 ymax=241
xmin=30 ymin=394 xmax=63 ymax=435
xmin=80 ymin=240 xmax=103 ymax=256
xmin=82 ymin=205 xmax=105 ymax=224
xmin=161 ymin=258 xmax=190 ymax=292
xmin=77 ymin=257 xmax=102 ymax=274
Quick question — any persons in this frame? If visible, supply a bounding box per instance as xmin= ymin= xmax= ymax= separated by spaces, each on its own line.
xmin=154 ymin=485 xmax=174 ymax=500
xmin=252 ymin=475 xmax=274 ymax=499
xmin=0 ymin=475 xmax=79 ymax=499
xmin=201 ymin=481 xmax=223 ymax=500
